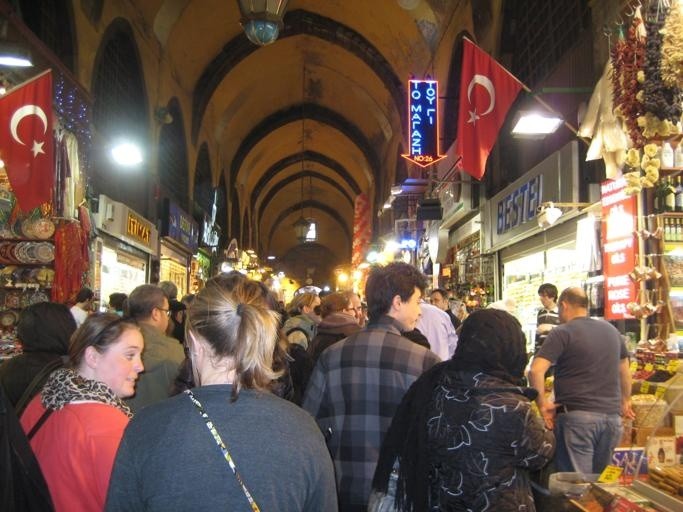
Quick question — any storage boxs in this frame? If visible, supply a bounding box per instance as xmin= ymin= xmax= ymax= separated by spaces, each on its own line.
xmin=631 ymin=394 xmax=668 ymax=432
xmin=631 ymin=353 xmax=683 ymax=415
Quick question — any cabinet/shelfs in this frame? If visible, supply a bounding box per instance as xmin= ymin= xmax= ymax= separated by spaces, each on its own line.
xmin=568 ymin=350 xmax=683 ymax=511
xmin=646 ymin=125 xmax=683 ymax=336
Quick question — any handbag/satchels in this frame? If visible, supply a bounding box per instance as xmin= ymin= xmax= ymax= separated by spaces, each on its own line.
xmin=366 ymin=457 xmax=401 ymax=512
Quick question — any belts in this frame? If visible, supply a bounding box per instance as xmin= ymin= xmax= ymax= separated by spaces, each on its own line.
xmin=555 ymin=405 xmax=572 ymax=413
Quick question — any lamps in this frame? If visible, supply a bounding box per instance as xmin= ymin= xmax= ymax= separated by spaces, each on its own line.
xmin=304 ymin=216 xmax=318 ymax=243
xmin=292 ymin=66 xmax=310 ymax=244
xmin=416 ymin=199 xmax=442 ymax=220
xmin=238 ymin=0 xmax=286 ymax=46
xmin=508 ymin=100 xmax=565 ymax=142
xmin=538 ymin=201 xmax=611 ymax=225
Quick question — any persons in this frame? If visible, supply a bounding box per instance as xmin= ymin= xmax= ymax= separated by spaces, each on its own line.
xmin=20 ymin=312 xmax=145 ymax=512
xmin=308 ymin=292 xmax=360 ymax=359
xmin=302 ymin=264 xmax=441 ymax=512
xmin=416 ymin=302 xmax=458 ymax=361
xmin=429 ymin=289 xmax=461 ymax=331
xmin=105 ymin=269 xmax=339 ymax=512
xmin=108 ymin=294 xmax=128 ymax=316
xmin=0 ymin=301 xmax=77 ymax=409
xmin=527 ymin=286 xmax=636 ymax=472
xmin=158 ymin=280 xmax=187 ymax=346
xmin=122 ymin=284 xmax=186 ymax=410
xmin=279 ymin=292 xmax=321 ymax=404
xmin=180 ymin=293 xmax=195 ymax=308
xmin=345 ymin=290 xmax=363 ymax=327
xmin=393 ymin=306 xmax=556 ymax=512
xmin=68 ymin=287 xmax=93 ymax=327
xmin=534 ymin=284 xmax=560 ymax=351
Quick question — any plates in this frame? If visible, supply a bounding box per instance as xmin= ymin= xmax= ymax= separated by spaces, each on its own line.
xmin=0 ymin=242 xmax=9 ymax=263
xmin=0 ymin=312 xmax=15 ymax=328
xmin=10 ymin=310 xmax=20 ymax=325
xmin=14 ymin=241 xmax=25 ymax=263
xmin=24 ymin=243 xmax=37 ymax=264
xmin=32 ymin=219 xmax=55 ymax=239
xmin=11 ymin=223 xmax=21 ymax=240
xmin=5 ymin=290 xmax=22 ymax=308
xmin=19 ymin=242 xmax=30 ymax=264
xmin=7 ymin=242 xmax=18 ymax=264
xmin=28 ymin=291 xmax=49 ymax=306
xmin=21 ymin=219 xmax=34 ymax=239
xmin=19 ymin=290 xmax=29 ymax=309
xmin=34 ymin=241 xmax=55 ymax=263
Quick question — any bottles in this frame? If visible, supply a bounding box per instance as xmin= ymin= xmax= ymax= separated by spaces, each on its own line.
xmin=676 ymin=218 xmax=681 ymax=242
xmin=660 ymin=177 xmax=665 ymax=212
xmin=675 ymin=176 xmax=683 ymax=212
xmin=670 ymin=218 xmax=676 ymax=242
xmin=681 ymin=219 xmax=683 ymax=242
xmin=664 ymin=218 xmax=670 ymax=242
xmin=653 ymin=181 xmax=660 ymax=213
xmin=664 ymin=175 xmax=675 ymax=212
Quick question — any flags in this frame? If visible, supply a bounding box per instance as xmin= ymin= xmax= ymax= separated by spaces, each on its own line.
xmin=0 ymin=70 xmax=56 ymax=214
xmin=454 ymin=40 xmax=523 ymax=181
